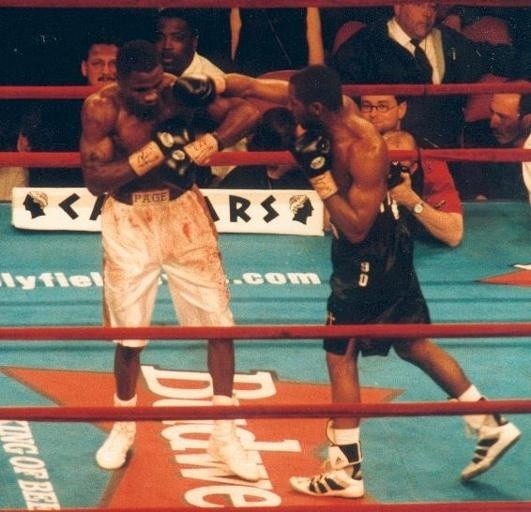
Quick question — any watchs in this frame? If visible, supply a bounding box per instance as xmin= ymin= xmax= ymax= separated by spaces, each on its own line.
xmin=411 ymin=202 xmax=426 ymax=216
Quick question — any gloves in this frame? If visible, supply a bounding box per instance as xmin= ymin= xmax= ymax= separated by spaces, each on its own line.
xmin=128 ymin=115 xmax=195 ymax=177
xmin=173 ymin=74 xmax=226 ymax=105
xmin=291 ymin=128 xmax=339 ymax=199
xmin=155 ymin=132 xmax=224 ymax=191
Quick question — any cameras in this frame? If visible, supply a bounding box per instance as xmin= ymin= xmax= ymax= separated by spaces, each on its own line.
xmin=388 ymin=160 xmax=410 ymax=189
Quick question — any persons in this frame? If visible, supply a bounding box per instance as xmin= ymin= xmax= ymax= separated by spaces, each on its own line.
xmin=173 ymin=61 xmax=521 ymax=500
xmin=76 ymin=34 xmax=263 ymax=472
xmin=2 ymin=2 xmax=530 ymax=251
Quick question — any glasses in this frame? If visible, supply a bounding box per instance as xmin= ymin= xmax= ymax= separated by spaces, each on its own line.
xmin=360 ymin=103 xmax=401 ymax=113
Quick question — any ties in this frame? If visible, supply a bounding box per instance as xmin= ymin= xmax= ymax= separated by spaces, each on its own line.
xmin=410 ymin=38 xmax=434 ymax=84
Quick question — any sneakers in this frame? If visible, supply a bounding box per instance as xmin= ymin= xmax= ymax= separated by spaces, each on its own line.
xmin=95 ymin=420 xmax=136 ymax=470
xmin=460 ymin=420 xmax=523 ymax=481
xmin=289 ymin=468 xmax=364 ymax=498
xmin=205 ymin=430 xmax=261 ymax=481
